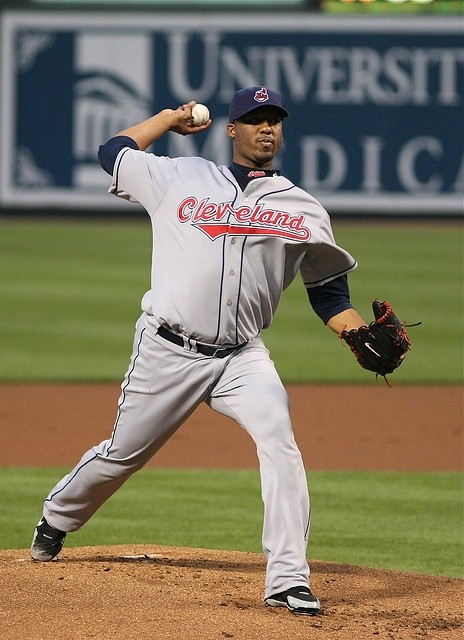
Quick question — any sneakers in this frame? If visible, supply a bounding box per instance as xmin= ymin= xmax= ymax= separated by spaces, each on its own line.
xmin=266 ymin=587 xmax=320 ymax=617
xmin=31 ymin=516 xmax=67 ymax=561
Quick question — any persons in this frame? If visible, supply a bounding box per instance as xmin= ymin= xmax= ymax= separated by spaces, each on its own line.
xmin=29 ymin=86 xmax=411 ymax=617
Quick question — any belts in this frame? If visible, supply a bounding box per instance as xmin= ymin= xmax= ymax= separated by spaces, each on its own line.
xmin=156 ymin=326 xmax=248 ymax=359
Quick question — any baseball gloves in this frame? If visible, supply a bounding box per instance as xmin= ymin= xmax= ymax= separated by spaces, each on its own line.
xmin=338 ymin=298 xmax=422 ymax=389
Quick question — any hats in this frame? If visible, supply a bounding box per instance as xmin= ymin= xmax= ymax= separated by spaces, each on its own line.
xmin=229 ymin=86 xmax=289 ymax=124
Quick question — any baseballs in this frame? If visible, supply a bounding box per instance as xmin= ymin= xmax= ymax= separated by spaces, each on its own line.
xmin=188 ymin=102 xmax=210 ymax=126
